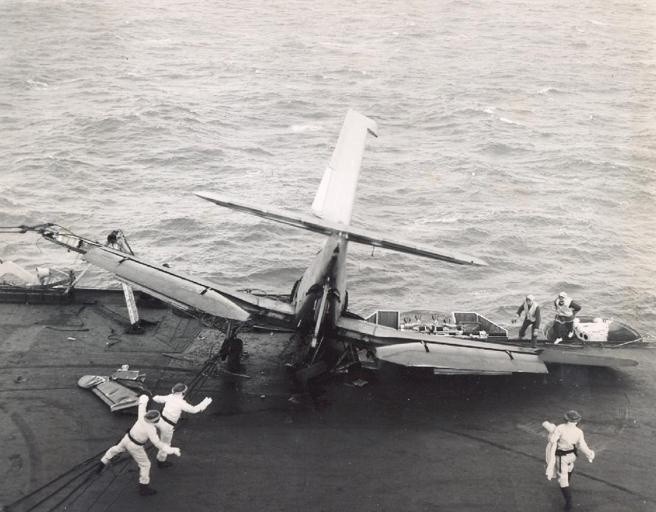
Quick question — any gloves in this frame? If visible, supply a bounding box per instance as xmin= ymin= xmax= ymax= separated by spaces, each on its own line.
xmin=199 ymin=396 xmax=212 ymax=412
xmin=164 ymin=445 xmax=180 ymax=457
xmin=511 ymin=314 xmax=520 ymax=324
xmin=139 ymin=393 xmax=150 ymax=405
xmin=533 ymin=329 xmax=539 ymax=337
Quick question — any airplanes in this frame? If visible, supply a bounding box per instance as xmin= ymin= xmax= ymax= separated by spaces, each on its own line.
xmin=18 ymin=104 xmax=644 ymax=413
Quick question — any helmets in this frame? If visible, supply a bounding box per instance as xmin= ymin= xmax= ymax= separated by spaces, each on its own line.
xmin=143 ymin=409 xmax=160 ymax=423
xmin=172 ymin=383 xmax=188 ymax=397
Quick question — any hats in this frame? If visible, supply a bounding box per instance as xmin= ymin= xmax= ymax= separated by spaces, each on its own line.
xmin=564 ymin=409 xmax=582 ymax=422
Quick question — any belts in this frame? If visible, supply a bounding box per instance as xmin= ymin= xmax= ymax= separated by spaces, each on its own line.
xmin=557 ymin=449 xmax=576 ymax=453
xmin=160 ymin=411 xmax=176 ymax=427
xmin=127 ymin=428 xmax=146 ymax=445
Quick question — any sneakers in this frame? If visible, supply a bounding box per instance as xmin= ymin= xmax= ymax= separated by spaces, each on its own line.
xmin=553 ymin=337 xmax=563 ymax=345
xmin=568 ymin=331 xmax=574 ymax=337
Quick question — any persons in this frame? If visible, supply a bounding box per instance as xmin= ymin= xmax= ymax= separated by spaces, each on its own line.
xmin=96 ymin=393 xmax=181 ymax=496
xmin=152 ymin=382 xmax=214 ymax=467
xmin=545 ymin=410 xmax=596 ymax=512
xmin=553 ymin=292 xmax=581 ymax=345
xmin=511 ymin=295 xmax=541 ymax=348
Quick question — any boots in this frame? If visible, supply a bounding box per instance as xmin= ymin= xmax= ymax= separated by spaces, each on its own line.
xmin=158 ymin=460 xmax=172 ymax=467
xmin=134 ymin=482 xmax=156 ymax=496
xmin=95 ymin=460 xmax=105 ymax=474
xmin=559 ymin=471 xmax=573 ymax=511
